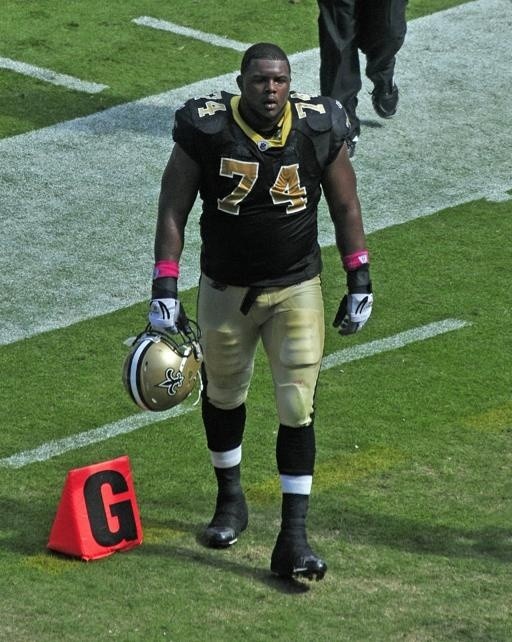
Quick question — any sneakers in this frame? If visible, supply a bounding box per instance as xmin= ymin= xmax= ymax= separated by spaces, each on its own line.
xmin=365 ymin=59 xmax=400 ymax=119
xmin=206 ymin=496 xmax=248 ymax=549
xmin=271 ymin=545 xmax=328 ymax=580
xmin=346 ymin=137 xmax=357 ymax=163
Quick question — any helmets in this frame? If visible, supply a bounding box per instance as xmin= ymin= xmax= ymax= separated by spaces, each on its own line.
xmin=123 ymin=318 xmax=202 ymax=411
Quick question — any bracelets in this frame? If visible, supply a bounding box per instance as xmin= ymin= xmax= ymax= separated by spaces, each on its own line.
xmin=153 ymin=260 xmax=180 ymax=280
xmin=342 ymin=249 xmax=369 ymax=273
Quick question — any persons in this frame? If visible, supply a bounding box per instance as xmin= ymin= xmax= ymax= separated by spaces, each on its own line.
xmin=149 ymin=42 xmax=373 ymax=589
xmin=315 ymin=2 xmax=406 ymax=162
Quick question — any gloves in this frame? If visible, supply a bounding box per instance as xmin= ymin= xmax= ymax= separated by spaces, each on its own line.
xmin=332 ymin=266 xmax=375 ymax=336
xmin=146 ymin=277 xmax=191 ymax=334
